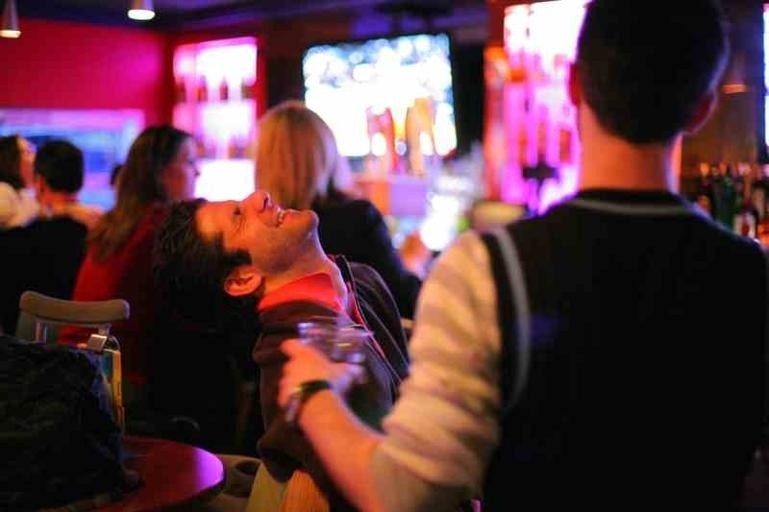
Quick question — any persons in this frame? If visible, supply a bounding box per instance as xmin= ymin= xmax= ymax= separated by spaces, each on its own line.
xmin=273 ymin=0 xmax=769 ymax=510
xmin=55 ymin=123 xmax=201 ymax=440
xmin=148 ymin=188 xmax=414 ymax=511
xmin=0 ymin=134 xmax=41 ymax=225
xmin=232 ymin=100 xmax=410 ymax=461
xmin=0 ymin=140 xmax=107 ymax=232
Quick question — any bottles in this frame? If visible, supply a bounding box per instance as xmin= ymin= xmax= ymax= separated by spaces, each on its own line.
xmin=196 ymin=133 xmax=247 ymax=158
xmin=693 ymin=159 xmax=769 ymax=246
xmin=197 ymin=76 xmax=208 ymax=104
xmin=220 ymin=78 xmax=228 ymax=101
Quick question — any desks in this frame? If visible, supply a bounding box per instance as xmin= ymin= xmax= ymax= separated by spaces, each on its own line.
xmin=39 ymin=433 xmax=228 ymax=512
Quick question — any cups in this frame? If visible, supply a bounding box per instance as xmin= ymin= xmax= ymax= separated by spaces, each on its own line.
xmin=296 ymin=322 xmax=375 ymax=424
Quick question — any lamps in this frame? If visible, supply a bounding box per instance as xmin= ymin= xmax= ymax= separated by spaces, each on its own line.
xmin=127 ymin=0 xmax=155 ymax=21
xmin=0 ymin=0 xmax=22 ymax=38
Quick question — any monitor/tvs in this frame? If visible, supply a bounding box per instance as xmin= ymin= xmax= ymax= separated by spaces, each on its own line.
xmin=300 ymin=33 xmax=470 ymax=168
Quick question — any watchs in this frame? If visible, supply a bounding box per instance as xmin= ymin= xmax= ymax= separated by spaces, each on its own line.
xmin=277 ymin=378 xmax=333 ymax=424
